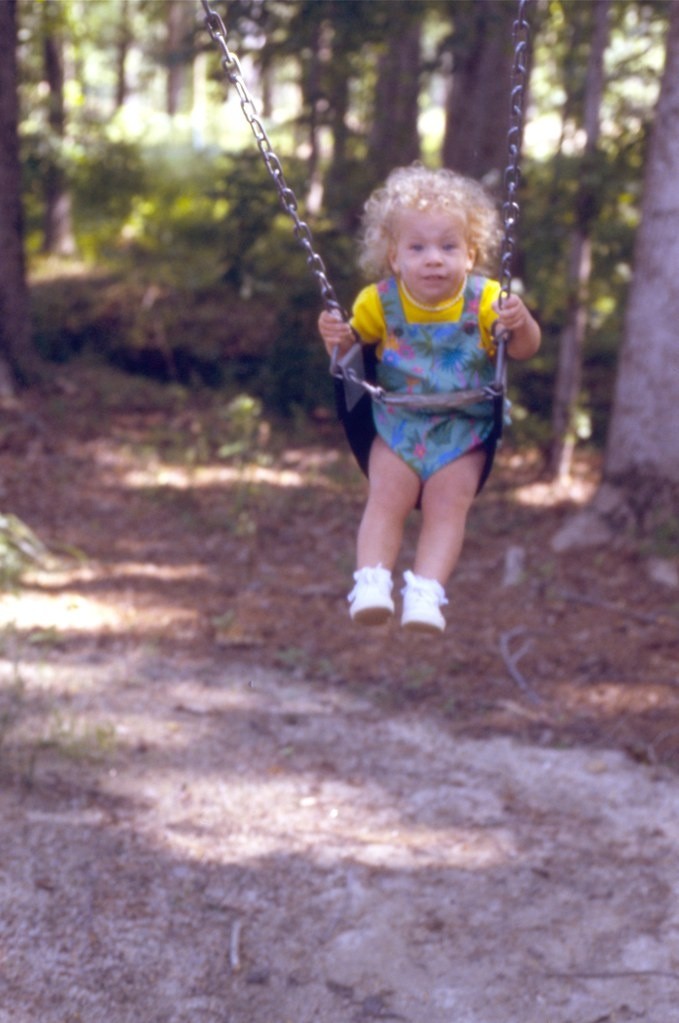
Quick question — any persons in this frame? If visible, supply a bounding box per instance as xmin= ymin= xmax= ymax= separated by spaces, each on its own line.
xmin=317 ymin=166 xmax=543 ymax=629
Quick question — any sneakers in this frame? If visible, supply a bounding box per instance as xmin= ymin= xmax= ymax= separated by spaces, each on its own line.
xmin=348 ymin=568 xmax=394 ymax=627
xmin=399 ymin=568 xmax=447 ymax=631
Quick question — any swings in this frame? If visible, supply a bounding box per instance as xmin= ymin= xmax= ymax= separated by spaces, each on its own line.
xmin=201 ymin=1 xmax=534 ymax=512
